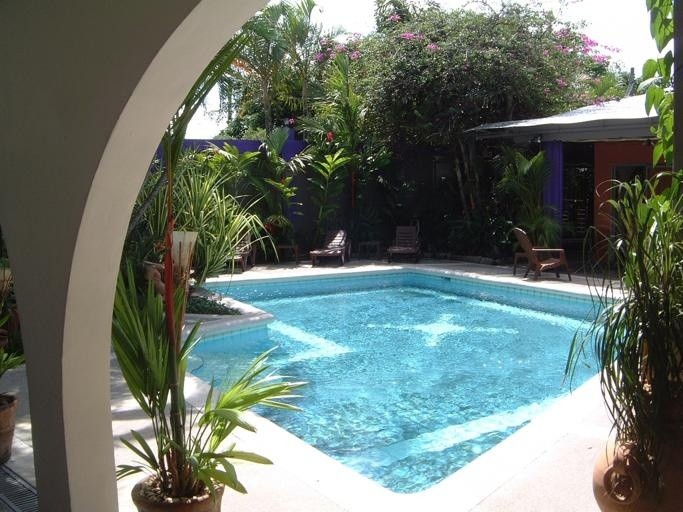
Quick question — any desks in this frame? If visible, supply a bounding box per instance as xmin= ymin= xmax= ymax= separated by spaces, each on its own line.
xmin=271 ymin=238 xmax=302 ymax=269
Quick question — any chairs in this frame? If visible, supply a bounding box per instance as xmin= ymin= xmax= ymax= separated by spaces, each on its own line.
xmin=512 ymin=227 xmax=571 ymax=281
xmin=380 ymin=214 xmax=423 ymax=265
xmin=218 ymin=219 xmax=253 ymax=272
xmin=308 ymin=224 xmax=352 ymax=267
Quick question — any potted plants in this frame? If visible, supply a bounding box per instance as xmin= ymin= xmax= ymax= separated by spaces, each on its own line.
xmin=0 ymin=254 xmax=32 ymax=463
xmin=104 ymin=38 xmax=310 ymax=512
xmin=564 ymin=167 xmax=682 ymax=512
